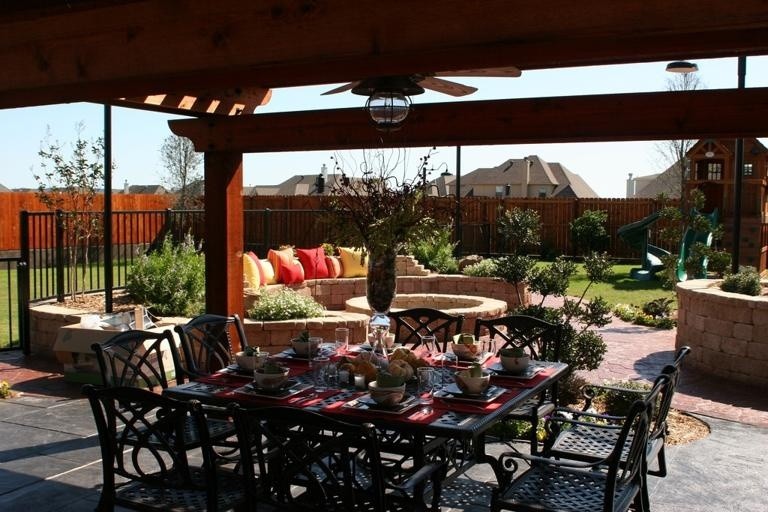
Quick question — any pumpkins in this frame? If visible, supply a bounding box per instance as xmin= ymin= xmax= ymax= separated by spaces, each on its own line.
xmin=389 ymin=348 xmax=417 ymax=366
xmin=387 ymin=358 xmax=413 ymax=382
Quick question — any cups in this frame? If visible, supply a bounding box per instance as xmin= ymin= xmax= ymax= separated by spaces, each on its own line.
xmin=335 ymin=328 xmax=349 ymax=351
xmin=338 ymin=369 xmax=349 ymax=386
xmin=421 ymin=336 xmax=435 ymax=352
xmin=312 ymin=357 xmax=330 ymax=392
xmin=353 ymin=368 xmax=366 ymax=391
xmin=253 ymin=352 xmax=269 ymax=371
xmin=308 ymin=337 xmax=323 ymax=368
xmin=442 ymin=355 xmax=458 ymax=384
xmin=481 ymin=339 xmax=496 ymax=358
xmin=122 ymin=312 xmax=131 ymax=329
xmin=416 ymin=366 xmax=440 ymax=405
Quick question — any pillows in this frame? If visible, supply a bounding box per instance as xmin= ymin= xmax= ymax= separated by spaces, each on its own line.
xmin=273 ymin=249 xmax=293 ymax=280
xmin=325 ymin=256 xmax=342 ymax=278
xmin=243 ymin=254 xmax=259 ymax=289
xmin=282 ymin=259 xmax=305 ymax=283
xmin=337 ymin=246 xmax=368 ymax=278
xmin=248 ymin=251 xmax=264 ymax=285
xmin=297 ymin=247 xmax=328 ymax=279
xmin=259 ymin=259 xmax=277 ymax=285
xmin=267 ymin=248 xmax=275 ymax=262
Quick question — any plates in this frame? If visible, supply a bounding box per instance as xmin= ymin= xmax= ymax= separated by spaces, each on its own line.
xmin=215 ymin=367 xmax=254 ymax=380
xmin=340 ymin=393 xmax=419 ymax=415
xmin=227 ymin=364 xmax=252 ymax=373
xmin=282 ymin=349 xmax=327 ymax=358
xmin=440 ymin=383 xmax=497 ymax=399
xmin=233 ymin=385 xmax=317 ymax=401
xmin=243 ymin=381 xmax=301 ymax=393
xmin=359 ymin=345 xmax=397 ymax=353
xmin=485 ymin=363 xmax=532 ymax=376
xmin=272 ymin=352 xmax=335 ymax=362
xmin=457 ymin=359 xmax=483 ymax=365
xmin=357 ymin=396 xmax=416 ymax=410
xmin=437 ymin=352 xmax=494 ymax=370
xmin=347 ymin=346 xmax=405 ymax=356
xmin=491 ymin=367 xmax=544 ymax=381
xmin=433 ymin=388 xmax=507 ymax=404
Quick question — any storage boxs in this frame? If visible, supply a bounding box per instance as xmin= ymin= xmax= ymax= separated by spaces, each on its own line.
xmin=52 ymin=319 xmax=178 ymax=387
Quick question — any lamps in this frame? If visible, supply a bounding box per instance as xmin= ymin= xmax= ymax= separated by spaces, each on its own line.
xmin=666 ymin=61 xmax=699 ymax=74
xmin=352 ymin=77 xmax=425 ymax=132
xmin=706 ymin=151 xmax=715 ymax=159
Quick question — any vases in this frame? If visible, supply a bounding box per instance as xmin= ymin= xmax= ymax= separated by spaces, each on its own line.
xmin=367 ymin=252 xmax=397 ymax=324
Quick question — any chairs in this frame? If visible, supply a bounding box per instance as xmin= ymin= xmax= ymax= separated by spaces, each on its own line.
xmin=232 ymin=401 xmax=447 ymax=511
xmin=387 ymin=307 xmax=466 ymax=353
xmin=489 ymin=375 xmax=671 ymax=512
xmin=83 ymin=383 xmax=239 ymax=512
xmin=473 ymin=315 xmax=565 ymax=464
xmin=174 ymin=312 xmax=271 ymax=458
xmin=548 ymin=346 xmax=691 ymax=477
xmin=90 ymin=330 xmax=239 ymax=472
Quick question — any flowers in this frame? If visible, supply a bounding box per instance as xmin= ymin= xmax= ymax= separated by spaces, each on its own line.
xmin=312 ymin=138 xmax=468 ymax=251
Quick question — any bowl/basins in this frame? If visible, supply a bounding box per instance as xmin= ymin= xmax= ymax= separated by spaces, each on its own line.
xmin=290 ymin=338 xmax=322 ymax=355
xmin=451 ymin=343 xmax=480 ymax=360
xmin=234 ymin=352 xmax=268 ymax=370
xmin=252 ymin=367 xmax=290 ymax=391
xmin=367 ymin=381 xmax=406 ymax=405
xmin=500 ymin=354 xmax=530 ymax=370
xmin=367 ymin=332 xmax=395 ymax=348
xmin=454 ymin=370 xmax=490 ymax=395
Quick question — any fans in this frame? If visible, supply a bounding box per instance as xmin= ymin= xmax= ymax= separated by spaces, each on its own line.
xmin=321 ymin=27 xmax=520 ymax=98
